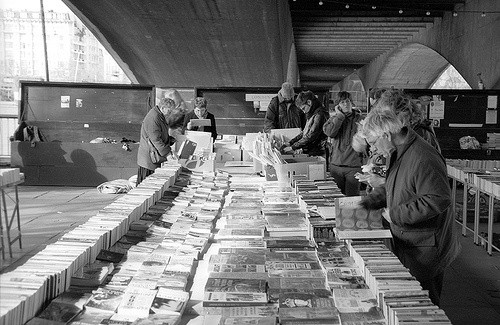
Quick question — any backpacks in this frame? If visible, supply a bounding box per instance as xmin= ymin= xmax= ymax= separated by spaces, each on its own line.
xmin=24 ymin=126 xmax=48 ymax=147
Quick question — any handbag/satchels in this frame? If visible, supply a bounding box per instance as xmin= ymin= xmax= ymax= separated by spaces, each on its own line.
xmin=148 ymin=138 xmax=167 ymax=165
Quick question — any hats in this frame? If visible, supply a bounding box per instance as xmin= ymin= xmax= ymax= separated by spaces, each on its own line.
xmin=281 ymin=82 xmax=295 ymax=98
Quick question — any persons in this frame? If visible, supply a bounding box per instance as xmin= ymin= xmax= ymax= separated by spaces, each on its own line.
xmin=136 ymin=89 xmax=218 ymax=188
xmin=264 ymin=83 xmax=462 ymax=309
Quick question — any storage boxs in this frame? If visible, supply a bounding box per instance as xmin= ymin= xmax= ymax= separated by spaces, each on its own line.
xmin=9 ymin=80 xmax=156 ymax=187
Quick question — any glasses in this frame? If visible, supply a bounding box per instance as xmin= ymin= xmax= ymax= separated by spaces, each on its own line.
xmin=367 ymin=136 xmax=380 ymax=148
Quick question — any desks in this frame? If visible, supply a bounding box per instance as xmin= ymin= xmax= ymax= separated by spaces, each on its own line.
xmin=0 ymin=173 xmax=24 ymax=260
xmin=447 ymin=159 xmax=500 ymax=256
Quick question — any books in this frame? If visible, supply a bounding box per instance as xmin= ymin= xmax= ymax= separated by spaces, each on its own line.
xmin=0 ymin=119 xmax=500 ymax=325
